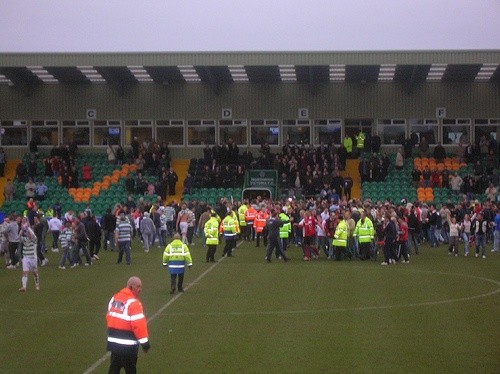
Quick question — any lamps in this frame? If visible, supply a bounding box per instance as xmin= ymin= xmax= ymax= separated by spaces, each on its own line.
xmin=0 ymin=63 xmax=500 ymax=86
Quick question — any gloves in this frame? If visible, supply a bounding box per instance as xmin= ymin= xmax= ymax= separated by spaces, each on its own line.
xmin=142 ymin=342 xmax=150 ymax=352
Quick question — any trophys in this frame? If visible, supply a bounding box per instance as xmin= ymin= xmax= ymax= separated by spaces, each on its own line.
xmin=22 ymin=216 xmax=30 ymax=238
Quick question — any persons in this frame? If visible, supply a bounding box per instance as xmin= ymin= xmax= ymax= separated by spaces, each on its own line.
xmin=0 ymin=126 xmax=500 ymax=294
xmin=105 ymin=276 xmax=150 ymax=374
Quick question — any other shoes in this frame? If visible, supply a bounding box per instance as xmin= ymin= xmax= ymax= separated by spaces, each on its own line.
xmin=170 ymin=289 xmax=184 ymax=294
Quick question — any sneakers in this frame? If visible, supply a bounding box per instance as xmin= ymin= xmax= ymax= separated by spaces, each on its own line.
xmin=18 ymin=288 xmax=26 ymax=291
xmin=0 ymin=229 xmax=500 ymax=270
xmin=35 ymin=284 xmax=40 ymax=290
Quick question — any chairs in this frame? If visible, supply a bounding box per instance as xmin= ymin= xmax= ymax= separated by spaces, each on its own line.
xmin=0 ymin=147 xmax=500 ymax=216
